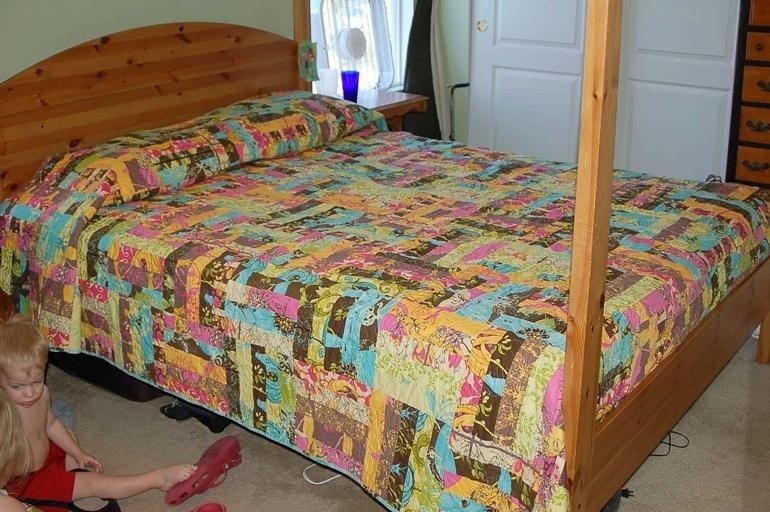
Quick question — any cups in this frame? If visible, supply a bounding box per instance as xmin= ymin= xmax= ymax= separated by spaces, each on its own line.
xmin=317 ymin=69 xmax=338 ymax=97
xmin=341 ymin=70 xmax=359 ymax=104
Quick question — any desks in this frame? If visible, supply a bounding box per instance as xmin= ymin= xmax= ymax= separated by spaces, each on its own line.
xmin=340 ymin=82 xmax=430 ymax=132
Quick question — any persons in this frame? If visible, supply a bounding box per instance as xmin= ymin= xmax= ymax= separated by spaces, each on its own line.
xmin=0 ymin=319 xmax=197 ymax=512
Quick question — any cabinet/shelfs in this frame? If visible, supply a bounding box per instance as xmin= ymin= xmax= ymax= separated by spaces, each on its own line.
xmin=723 ymin=0 xmax=770 ymax=184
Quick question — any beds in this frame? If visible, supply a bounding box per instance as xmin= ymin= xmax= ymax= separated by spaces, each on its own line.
xmin=0 ymin=24 xmax=768 ymax=508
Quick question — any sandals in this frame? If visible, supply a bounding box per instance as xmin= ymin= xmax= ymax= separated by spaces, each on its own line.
xmin=164 ymin=435 xmax=242 ymax=512
